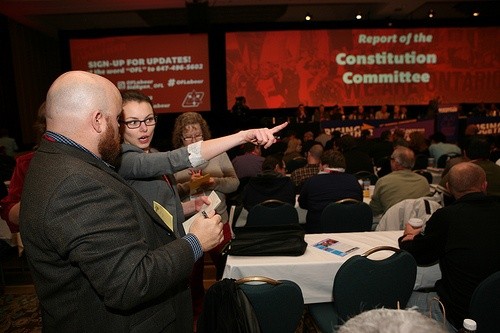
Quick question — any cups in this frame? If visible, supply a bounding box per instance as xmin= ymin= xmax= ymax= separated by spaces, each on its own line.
xmin=407 ymin=217 xmax=423 ymax=230
xmin=362 ymin=181 xmax=370 ymax=196
xmin=428 ymin=158 xmax=435 ymax=168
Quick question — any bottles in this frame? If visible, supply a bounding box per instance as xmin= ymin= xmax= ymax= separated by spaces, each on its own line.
xmin=461 ymin=318 xmax=478 ymax=333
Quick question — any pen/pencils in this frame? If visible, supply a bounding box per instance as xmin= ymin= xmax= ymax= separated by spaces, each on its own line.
xmin=251 ymin=136 xmax=280 ymax=143
xmin=202 ymin=211 xmax=209 ymax=219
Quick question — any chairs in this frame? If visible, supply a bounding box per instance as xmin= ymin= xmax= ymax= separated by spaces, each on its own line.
xmin=198 ymin=155 xmax=500 ymax=333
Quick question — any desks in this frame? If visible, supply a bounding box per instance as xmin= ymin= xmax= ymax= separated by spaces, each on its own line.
xmin=228 ymin=185 xmax=383 ymax=239
xmin=221 ymin=230 xmax=442 ymax=306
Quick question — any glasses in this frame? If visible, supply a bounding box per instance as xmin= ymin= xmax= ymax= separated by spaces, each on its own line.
xmin=120 ymin=115 xmax=157 ymax=129
xmin=181 ymin=134 xmax=204 ymax=140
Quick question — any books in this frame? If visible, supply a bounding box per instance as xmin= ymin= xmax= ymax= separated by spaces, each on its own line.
xmin=182 ymin=191 xmax=223 ymax=235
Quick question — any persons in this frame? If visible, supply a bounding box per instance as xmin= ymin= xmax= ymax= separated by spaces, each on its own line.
xmin=394 ymin=106 xmax=408 ymax=119
xmin=290 ymin=145 xmax=327 ymax=187
xmin=295 ymin=103 xmax=312 ymax=121
xmin=242 ymin=155 xmax=298 ymax=213
xmin=112 ymin=91 xmax=289 ymax=240
xmin=0 ymin=70 xmax=225 ymax=333
xmin=231 ymin=140 xmax=266 ymax=178
xmin=331 ymin=105 xmax=349 ymax=120
xmin=317 ymin=102 xmax=330 ymax=120
xmin=284 ymin=126 xmax=461 ymax=185
xmin=335 ymin=307 xmax=450 ymax=333
xmin=368 ymin=146 xmax=428 ymax=215
xmin=170 ymin=111 xmax=240 ymax=305
xmin=353 ymin=105 xmax=370 ymax=120
xmin=398 ymin=163 xmax=500 ymax=333
xmin=234 ymin=95 xmax=249 ymax=110
xmin=374 ymin=105 xmax=390 ymax=119
xmin=299 ymin=150 xmax=362 ymax=233
xmin=444 ymin=135 xmax=500 ymax=198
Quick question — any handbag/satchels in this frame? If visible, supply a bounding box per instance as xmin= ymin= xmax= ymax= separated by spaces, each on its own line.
xmin=218 ymin=226 xmax=307 ymax=256
